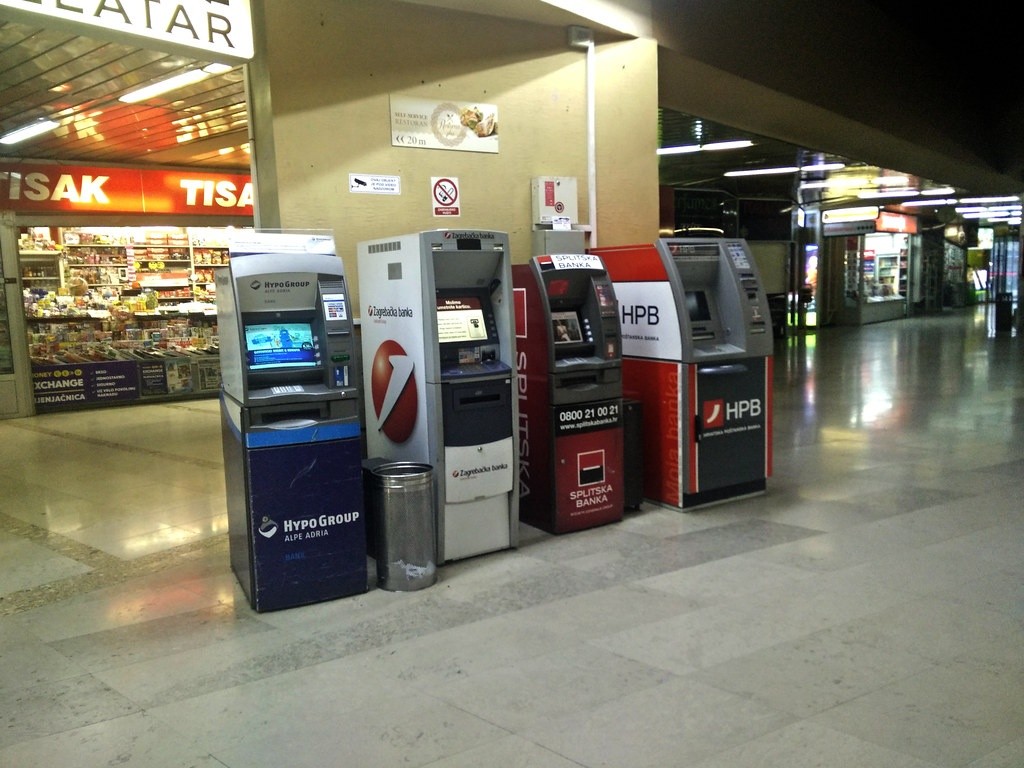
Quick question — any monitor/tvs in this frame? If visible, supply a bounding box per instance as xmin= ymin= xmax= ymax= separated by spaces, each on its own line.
xmin=245 ymin=323 xmax=317 ymax=370
xmin=551 ymin=307 xmax=588 ymax=344
xmin=684 ymin=291 xmax=711 ymax=322
xmin=436 ymin=296 xmax=489 ymax=344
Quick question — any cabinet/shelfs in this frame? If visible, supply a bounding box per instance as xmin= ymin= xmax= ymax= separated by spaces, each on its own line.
xmin=864 ymin=252 xmax=907 ymax=300
xmin=21 ymin=244 xmax=234 ymax=414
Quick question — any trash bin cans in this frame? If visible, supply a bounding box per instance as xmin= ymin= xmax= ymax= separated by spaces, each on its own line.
xmin=371 ymin=460 xmax=438 ymax=592
xmin=995 ymin=292 xmax=1013 ymax=333
